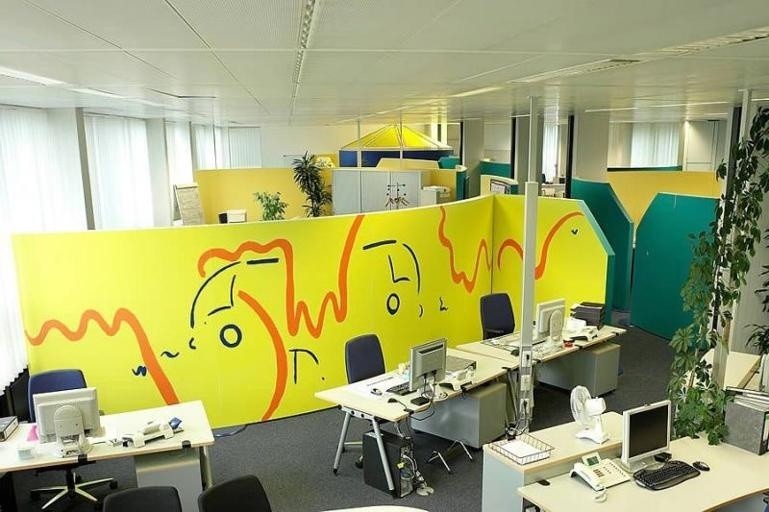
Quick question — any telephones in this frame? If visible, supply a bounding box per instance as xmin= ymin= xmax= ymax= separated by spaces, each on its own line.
xmin=434 ymin=368 xmax=474 ymax=391
xmin=568 ymin=451 xmax=631 ymax=492
xmin=569 ymin=325 xmax=599 ymax=341
xmin=122 ymin=420 xmax=174 ymax=447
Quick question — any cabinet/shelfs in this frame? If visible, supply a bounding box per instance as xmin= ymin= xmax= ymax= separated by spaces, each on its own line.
xmin=332 ymin=169 xmax=430 ymax=215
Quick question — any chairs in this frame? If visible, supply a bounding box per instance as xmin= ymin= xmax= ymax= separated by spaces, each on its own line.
xmin=102 ymin=485 xmax=184 ymax=512
xmin=198 ymin=475 xmax=272 ymax=512
xmin=480 ymin=294 xmax=515 ymax=340
xmin=28 ymin=369 xmax=118 ymax=512
xmin=338 ymin=334 xmax=386 ymax=468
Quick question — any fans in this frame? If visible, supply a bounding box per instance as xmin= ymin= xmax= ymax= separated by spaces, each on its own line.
xmin=570 ymin=385 xmax=611 ymax=443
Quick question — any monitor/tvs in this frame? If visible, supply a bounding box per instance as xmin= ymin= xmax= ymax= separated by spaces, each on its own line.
xmin=537 ymin=299 xmax=565 ymax=350
xmin=409 ymin=338 xmax=449 ymax=402
xmin=489 ymin=182 xmax=506 ymax=195
xmin=620 ymin=400 xmax=671 ymax=473
xmin=32 ymin=387 xmax=106 ymax=458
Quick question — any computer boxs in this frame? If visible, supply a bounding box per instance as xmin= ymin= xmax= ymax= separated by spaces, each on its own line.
xmin=362 ymin=429 xmax=415 ymax=499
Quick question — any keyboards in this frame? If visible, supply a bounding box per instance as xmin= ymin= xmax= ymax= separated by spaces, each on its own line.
xmin=506 ymin=336 xmax=545 ymax=348
xmin=385 ymin=382 xmax=417 ymax=396
xmin=633 ymin=460 xmax=700 ymax=490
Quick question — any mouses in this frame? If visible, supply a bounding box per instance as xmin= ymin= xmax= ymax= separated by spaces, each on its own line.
xmin=370 ymin=388 xmax=382 ymax=395
xmin=491 ymin=338 xmax=499 ymax=345
xmin=693 ymin=461 xmax=710 ymax=471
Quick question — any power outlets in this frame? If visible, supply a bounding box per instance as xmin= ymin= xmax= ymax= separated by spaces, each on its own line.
xmin=520 ymin=351 xmax=532 ymax=416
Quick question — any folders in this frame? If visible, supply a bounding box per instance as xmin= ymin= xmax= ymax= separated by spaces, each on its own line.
xmin=1 ymin=415 xmax=20 ymax=441
xmin=446 ymin=355 xmax=476 ymax=372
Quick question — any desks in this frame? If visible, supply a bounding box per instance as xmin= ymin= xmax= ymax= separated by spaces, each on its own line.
xmin=315 ymin=346 xmax=519 ymax=499
xmin=481 ymin=411 xmax=623 ymax=512
xmin=1 ymin=399 xmax=215 ymax=491
xmin=670 ymin=347 xmax=763 ymax=438
xmin=456 ymin=324 xmax=627 ymax=367
xmin=517 ymin=431 xmax=769 ymax=512
